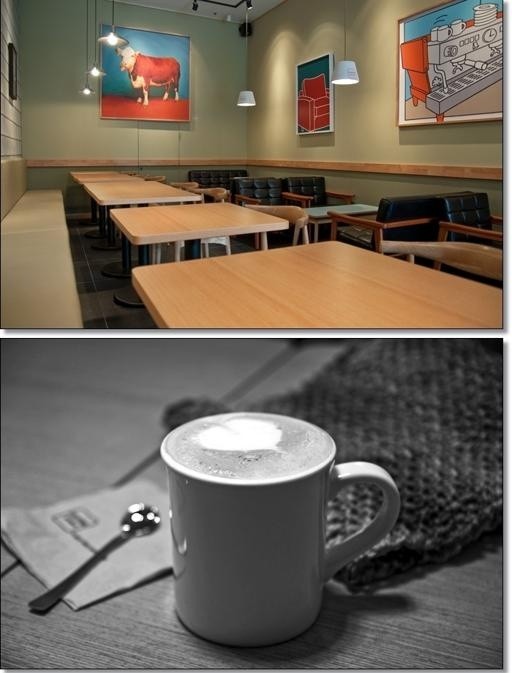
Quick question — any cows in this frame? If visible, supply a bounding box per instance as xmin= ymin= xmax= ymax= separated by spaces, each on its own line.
xmin=116 ymin=46 xmax=181 ymax=110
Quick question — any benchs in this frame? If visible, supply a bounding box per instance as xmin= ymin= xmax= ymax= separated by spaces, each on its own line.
xmin=1 ymin=153 xmax=85 ymax=329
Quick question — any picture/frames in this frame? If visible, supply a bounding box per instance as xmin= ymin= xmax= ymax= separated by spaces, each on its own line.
xmin=99 ymin=22 xmax=192 ymax=121
xmin=9 ymin=42 xmax=18 ymax=98
xmin=296 ymin=48 xmax=334 ymax=136
xmin=396 ymin=0 xmax=502 ymax=128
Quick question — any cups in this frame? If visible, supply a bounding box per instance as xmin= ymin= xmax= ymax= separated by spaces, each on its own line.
xmin=158 ymin=411 xmax=401 ymax=651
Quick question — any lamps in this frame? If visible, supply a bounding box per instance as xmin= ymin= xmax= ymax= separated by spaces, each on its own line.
xmin=191 ymin=0 xmax=253 ymax=11
xmin=79 ymin=0 xmax=95 ymax=95
xmin=237 ymin=5 xmax=256 ymax=106
xmin=85 ymin=0 xmax=107 ymax=78
xmin=330 ymin=0 xmax=360 ymax=85
xmin=98 ymin=0 xmax=129 ymax=49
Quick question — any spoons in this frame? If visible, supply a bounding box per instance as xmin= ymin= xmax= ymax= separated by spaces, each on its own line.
xmin=27 ymin=503 xmax=162 ymax=613
